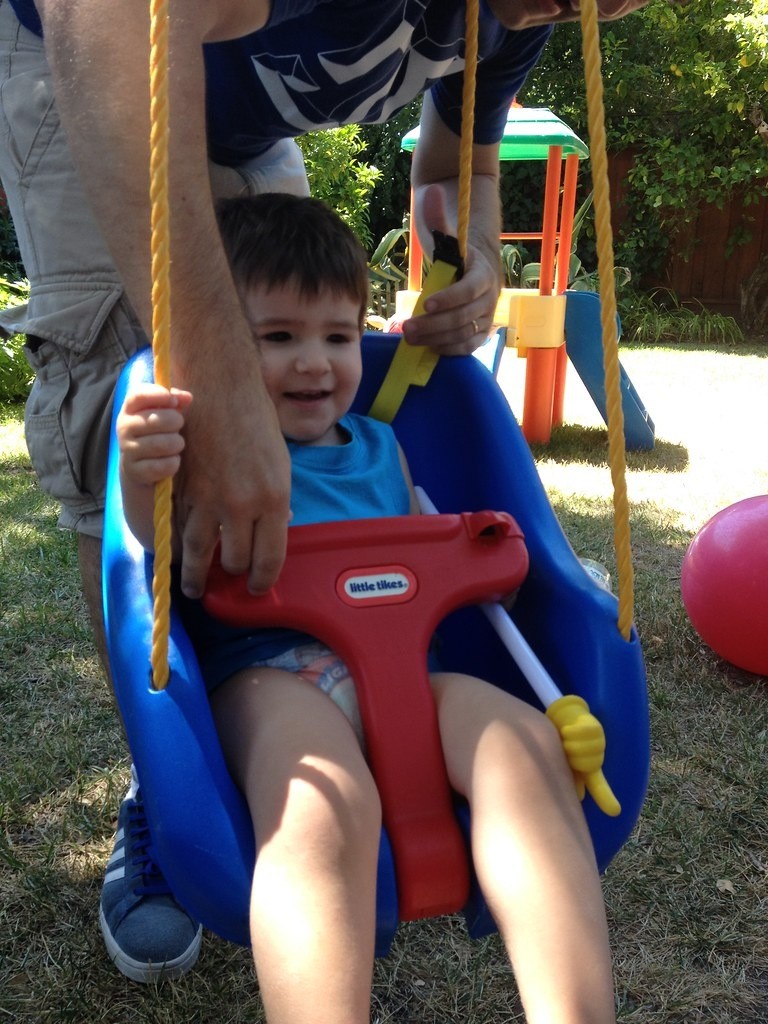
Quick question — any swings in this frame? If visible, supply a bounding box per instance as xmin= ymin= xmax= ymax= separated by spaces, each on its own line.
xmin=96 ymin=0 xmax=659 ymax=951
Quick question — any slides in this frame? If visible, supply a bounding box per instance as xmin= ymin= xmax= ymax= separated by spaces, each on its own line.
xmin=561 ymin=286 xmax=666 ymax=460
xmin=476 ymin=318 xmax=506 ymax=366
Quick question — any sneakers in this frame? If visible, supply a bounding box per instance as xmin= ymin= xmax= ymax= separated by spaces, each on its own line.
xmin=99 ymin=763 xmax=204 ymax=987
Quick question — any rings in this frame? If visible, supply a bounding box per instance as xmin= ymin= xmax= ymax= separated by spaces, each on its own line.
xmin=468 ymin=319 xmax=479 ymax=334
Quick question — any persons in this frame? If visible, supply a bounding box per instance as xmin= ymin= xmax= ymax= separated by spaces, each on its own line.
xmin=118 ymin=192 xmax=615 ymax=1024
xmin=3 ymin=0 xmax=654 ymax=986
